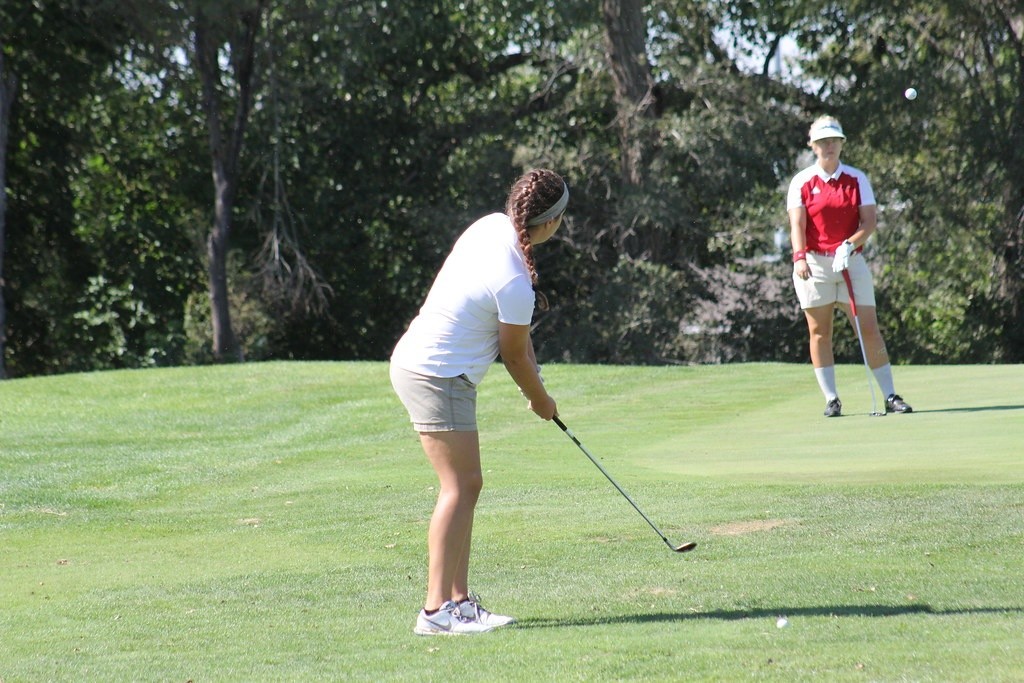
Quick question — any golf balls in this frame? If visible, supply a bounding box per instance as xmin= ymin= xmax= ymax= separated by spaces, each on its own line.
xmin=904 ymin=88 xmax=918 ymax=100
xmin=776 ymin=619 xmax=789 ymax=629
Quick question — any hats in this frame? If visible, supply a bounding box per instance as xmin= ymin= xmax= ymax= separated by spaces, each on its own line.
xmin=810 ymin=116 xmax=846 ymax=143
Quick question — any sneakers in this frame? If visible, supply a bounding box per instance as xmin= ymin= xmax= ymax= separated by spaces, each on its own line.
xmin=452 ymin=590 xmax=516 ymax=627
xmin=885 ymin=393 xmax=912 ymax=413
xmin=413 ymin=600 xmax=496 ymax=637
xmin=824 ymin=397 xmax=842 ymax=416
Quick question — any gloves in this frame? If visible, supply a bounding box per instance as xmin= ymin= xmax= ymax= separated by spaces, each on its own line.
xmin=832 ymin=239 xmax=855 ymax=273
xmin=517 ymin=364 xmax=544 ymax=400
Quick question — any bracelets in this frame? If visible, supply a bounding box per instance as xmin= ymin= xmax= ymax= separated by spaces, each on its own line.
xmin=793 ymin=250 xmax=806 ymax=263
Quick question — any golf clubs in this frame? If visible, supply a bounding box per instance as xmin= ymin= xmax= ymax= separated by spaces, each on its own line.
xmin=552 ymin=413 xmax=697 ymax=552
xmin=842 ymin=267 xmax=883 ymax=416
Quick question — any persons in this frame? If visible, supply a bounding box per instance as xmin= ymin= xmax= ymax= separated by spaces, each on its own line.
xmin=787 ymin=116 xmax=912 ymax=417
xmin=389 ymin=168 xmax=570 ymax=635
